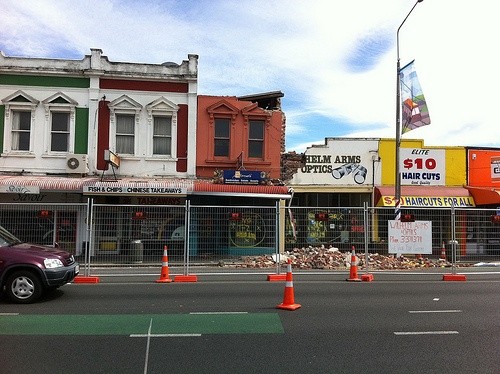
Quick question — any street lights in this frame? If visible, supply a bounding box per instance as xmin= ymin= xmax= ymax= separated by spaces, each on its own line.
xmin=395 ymin=0 xmax=424 ymax=260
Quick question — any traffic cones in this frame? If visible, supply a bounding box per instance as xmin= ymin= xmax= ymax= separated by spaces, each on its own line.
xmin=276 ymin=259 xmax=302 ymax=311
xmin=346 ymin=245 xmax=363 ymax=282
xmin=440 ymin=241 xmax=446 ymax=263
xmin=155 ymin=244 xmax=173 ymax=283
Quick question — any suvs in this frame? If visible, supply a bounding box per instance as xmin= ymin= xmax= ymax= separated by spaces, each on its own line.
xmin=0 ymin=225 xmax=80 ymax=304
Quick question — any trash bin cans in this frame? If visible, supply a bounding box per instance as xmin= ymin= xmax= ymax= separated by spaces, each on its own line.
xmin=445 ymin=240 xmax=459 ymax=262
xmin=130 ymin=240 xmax=143 ymax=264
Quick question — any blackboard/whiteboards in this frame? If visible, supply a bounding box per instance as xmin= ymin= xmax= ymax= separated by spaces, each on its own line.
xmin=387 ymin=220 xmax=432 ymax=255
